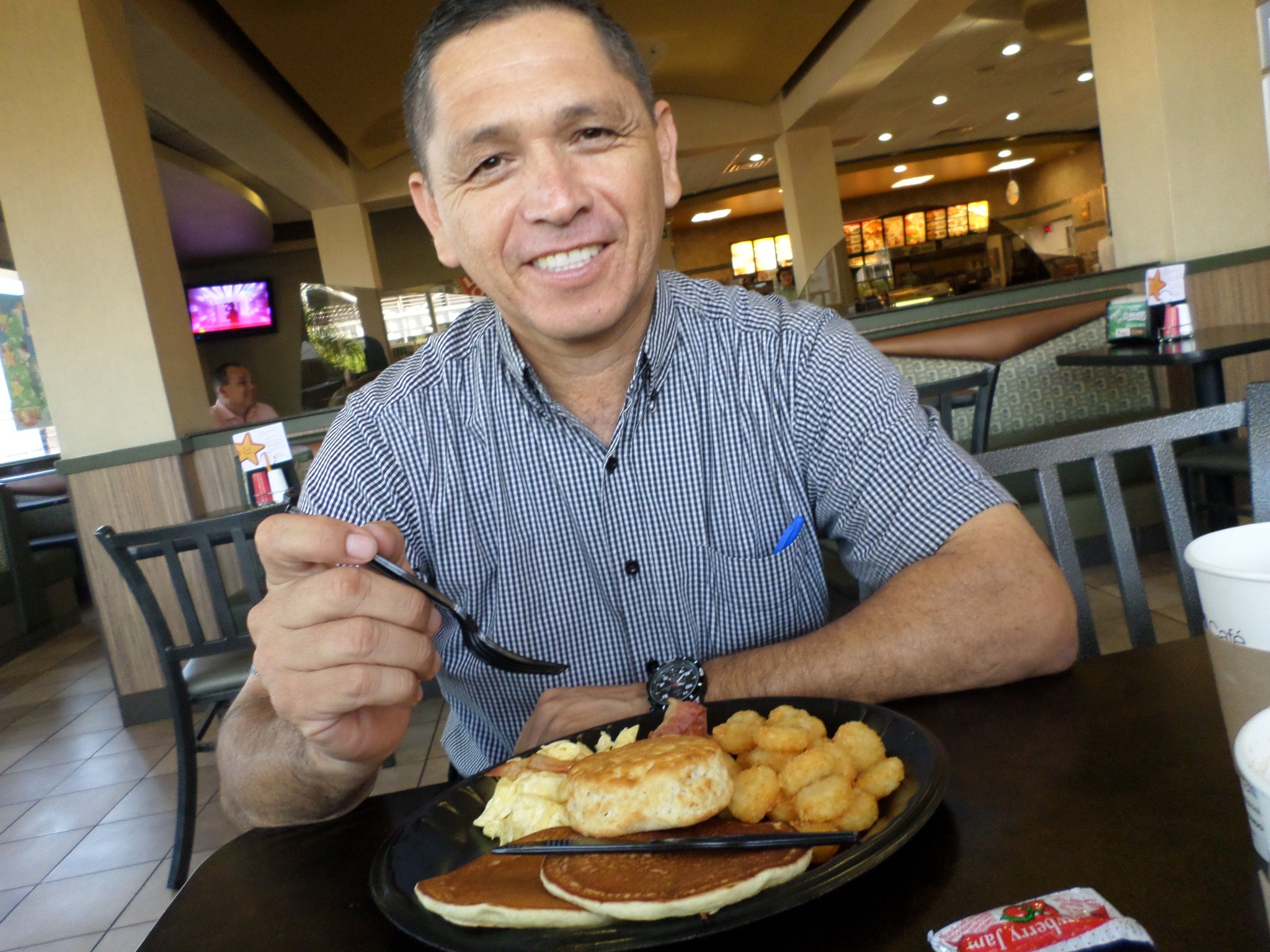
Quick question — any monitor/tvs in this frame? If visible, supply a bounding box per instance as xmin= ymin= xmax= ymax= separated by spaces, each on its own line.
xmin=181 ymin=277 xmax=278 ymax=341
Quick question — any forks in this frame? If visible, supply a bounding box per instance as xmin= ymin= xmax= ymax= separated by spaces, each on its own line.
xmin=285 ymin=504 xmax=571 ymax=676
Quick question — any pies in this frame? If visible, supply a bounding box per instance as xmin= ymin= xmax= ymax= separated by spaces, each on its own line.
xmin=414 ymin=736 xmax=812 ymax=929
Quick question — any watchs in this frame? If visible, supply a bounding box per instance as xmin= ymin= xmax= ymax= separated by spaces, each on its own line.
xmin=645 ymin=655 xmax=708 ymax=713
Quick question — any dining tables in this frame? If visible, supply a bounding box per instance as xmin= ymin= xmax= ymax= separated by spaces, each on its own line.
xmin=135 ymin=637 xmax=1270 ymax=952
xmin=1057 ymin=323 xmax=1270 ymax=539
xmin=125 ymin=502 xmax=399 ymax=770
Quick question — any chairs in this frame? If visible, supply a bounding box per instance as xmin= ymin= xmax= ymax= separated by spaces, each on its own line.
xmin=912 ymin=365 xmax=1000 ymax=454
xmin=966 ymin=400 xmax=1247 ymax=662
xmin=95 ymin=486 xmax=301 ymax=888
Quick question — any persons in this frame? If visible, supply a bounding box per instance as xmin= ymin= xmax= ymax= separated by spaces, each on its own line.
xmin=208 ymin=360 xmax=280 ymax=433
xmin=331 ymin=335 xmax=392 ymax=408
xmin=213 ymin=1 xmax=1081 ymax=828
xmin=772 ymin=267 xmax=802 ymax=303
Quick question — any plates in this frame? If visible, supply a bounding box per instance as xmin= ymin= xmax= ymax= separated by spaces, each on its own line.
xmin=366 ymin=697 xmax=950 ymax=952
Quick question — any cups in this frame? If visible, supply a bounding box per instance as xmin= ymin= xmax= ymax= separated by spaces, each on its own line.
xmin=1182 ymin=518 xmax=1270 ymax=931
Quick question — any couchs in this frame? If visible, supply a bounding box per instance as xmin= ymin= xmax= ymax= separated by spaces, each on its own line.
xmin=858 ymin=290 xmax=1173 ymax=556
xmin=0 ymin=468 xmax=93 ymax=667
